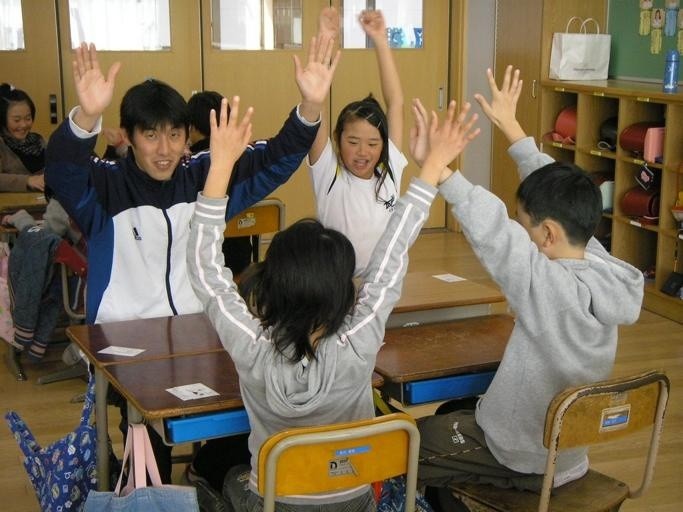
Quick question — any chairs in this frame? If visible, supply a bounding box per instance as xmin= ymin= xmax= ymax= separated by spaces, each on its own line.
xmin=253 ymin=411 xmax=423 ymax=511
xmin=445 ymin=367 xmax=670 ymax=511
xmin=35 ymin=267 xmax=102 ymax=388
xmin=220 ymin=198 xmax=286 ymax=288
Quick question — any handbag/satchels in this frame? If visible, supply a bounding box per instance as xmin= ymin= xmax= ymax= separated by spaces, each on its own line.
xmin=545 ymin=13 xmax=614 ymax=84
xmin=3 ymin=368 xmax=102 ymax=512
xmin=76 ymin=420 xmax=204 ymax=512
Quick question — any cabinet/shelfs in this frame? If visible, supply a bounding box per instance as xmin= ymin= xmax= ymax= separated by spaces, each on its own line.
xmin=539 ymin=79 xmax=682 ymax=326
xmin=489 ymin=1 xmax=612 ymax=228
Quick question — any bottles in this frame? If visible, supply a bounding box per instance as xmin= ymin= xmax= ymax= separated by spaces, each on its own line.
xmin=661 ymin=49 xmax=680 ymax=95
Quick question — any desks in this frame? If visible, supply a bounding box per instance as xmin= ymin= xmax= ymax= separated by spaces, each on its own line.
xmin=1 ymin=185 xmax=77 ymax=216
xmin=0 ymin=217 xmax=46 ymax=384
xmin=58 ymin=306 xmax=229 ymax=512
xmin=100 ymin=347 xmax=386 ymax=452
xmin=382 ymin=265 xmax=511 ymax=327
xmin=368 ymin=310 xmax=522 ymax=410
